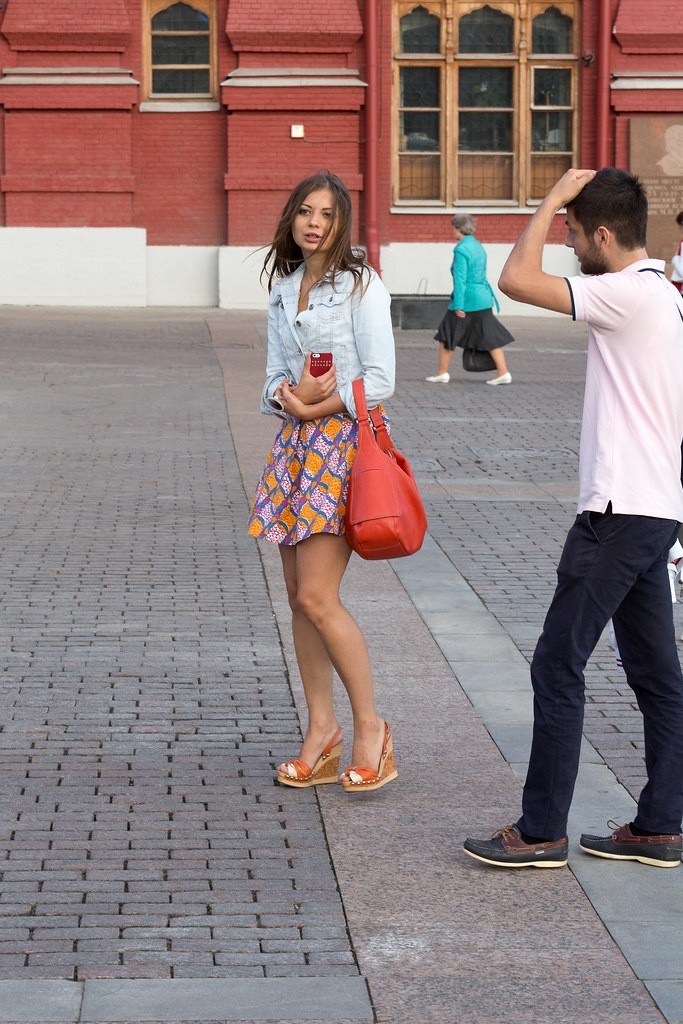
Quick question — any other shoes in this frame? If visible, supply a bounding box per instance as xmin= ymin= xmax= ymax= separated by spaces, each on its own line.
xmin=427 ymin=372 xmax=449 ymax=383
xmin=485 ymin=372 xmax=512 ymax=386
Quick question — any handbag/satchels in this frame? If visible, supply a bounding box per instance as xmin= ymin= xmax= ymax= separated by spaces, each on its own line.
xmin=346 ymin=379 xmax=427 ymax=560
xmin=462 ymin=349 xmax=497 ymax=372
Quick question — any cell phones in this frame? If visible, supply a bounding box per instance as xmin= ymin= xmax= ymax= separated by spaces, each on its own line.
xmin=310 ymin=352 xmax=333 ymax=378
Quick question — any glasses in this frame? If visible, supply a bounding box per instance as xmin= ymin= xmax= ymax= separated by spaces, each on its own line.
xmin=265 ymin=391 xmax=287 ymax=421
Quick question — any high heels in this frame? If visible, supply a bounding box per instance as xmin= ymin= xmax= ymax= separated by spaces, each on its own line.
xmin=277 ymin=726 xmax=343 ymax=787
xmin=342 ymin=722 xmax=398 ymax=791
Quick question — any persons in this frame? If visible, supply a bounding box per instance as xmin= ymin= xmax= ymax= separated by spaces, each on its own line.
xmin=424 ymin=212 xmax=515 ymax=385
xmin=246 ymin=167 xmax=399 ymax=792
xmin=458 ymin=167 xmax=682 ymax=869
xmin=668 ymin=210 xmax=683 ymax=297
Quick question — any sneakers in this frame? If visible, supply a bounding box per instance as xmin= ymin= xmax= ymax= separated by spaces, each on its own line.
xmin=463 ymin=823 xmax=569 ymax=868
xmin=579 ymin=820 xmax=682 ymax=867
